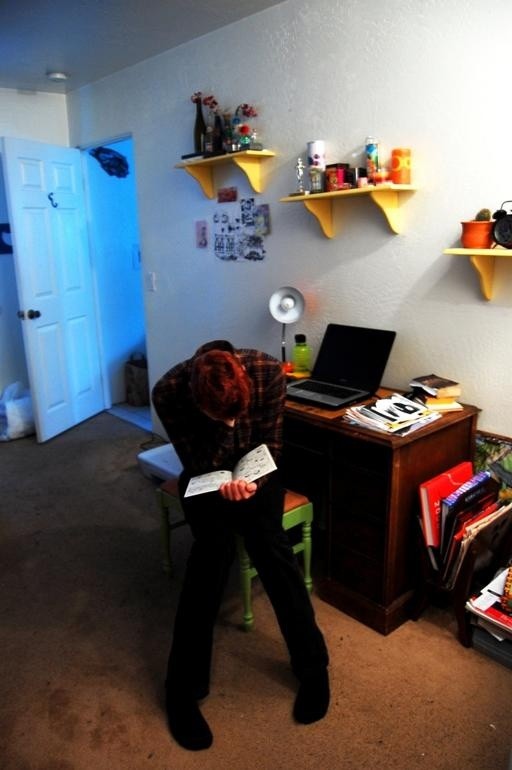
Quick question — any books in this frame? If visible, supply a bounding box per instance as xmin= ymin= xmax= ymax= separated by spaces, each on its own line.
xmin=339 ymin=375 xmax=464 ymax=438
xmin=182 ymin=443 xmax=277 ymax=500
xmin=415 ymin=460 xmax=511 ymax=666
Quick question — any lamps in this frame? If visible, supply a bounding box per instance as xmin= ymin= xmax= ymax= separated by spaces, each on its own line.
xmin=268 ymin=286 xmax=306 ymax=381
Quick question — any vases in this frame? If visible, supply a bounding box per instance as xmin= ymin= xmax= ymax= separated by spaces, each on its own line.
xmin=210 ymin=113 xmax=241 ymax=149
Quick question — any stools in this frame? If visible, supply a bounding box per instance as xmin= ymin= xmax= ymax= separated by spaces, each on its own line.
xmin=155 ymin=474 xmax=315 ymax=631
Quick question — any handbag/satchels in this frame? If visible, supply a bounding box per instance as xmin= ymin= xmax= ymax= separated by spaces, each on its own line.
xmin=124 ymin=351 xmax=151 ymax=407
xmin=0 ymin=381 xmax=38 ymax=441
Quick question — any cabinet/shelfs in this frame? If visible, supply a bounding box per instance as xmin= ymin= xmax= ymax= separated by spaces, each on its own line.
xmin=279 ymin=186 xmax=420 ymax=240
xmin=170 ymin=150 xmax=278 ymax=196
xmin=442 ymin=247 xmax=511 ymax=301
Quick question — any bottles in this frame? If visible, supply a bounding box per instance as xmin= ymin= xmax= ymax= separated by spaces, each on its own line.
xmin=323 ymin=163 xmax=349 ymax=191
xmin=292 ymin=332 xmax=313 ymax=373
xmin=194 ymin=100 xmax=230 ymax=155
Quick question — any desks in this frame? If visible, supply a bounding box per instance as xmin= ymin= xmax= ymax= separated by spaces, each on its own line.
xmin=279 ymin=370 xmax=482 ymax=635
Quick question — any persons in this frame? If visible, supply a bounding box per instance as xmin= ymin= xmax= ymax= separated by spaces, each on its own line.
xmin=150 ymin=339 xmax=333 ymax=753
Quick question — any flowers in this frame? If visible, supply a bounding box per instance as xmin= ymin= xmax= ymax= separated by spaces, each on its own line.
xmin=202 ymin=94 xmax=259 ymax=119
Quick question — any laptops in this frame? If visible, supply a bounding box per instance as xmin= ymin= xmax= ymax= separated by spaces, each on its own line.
xmin=285 ymin=323 xmax=396 ymax=411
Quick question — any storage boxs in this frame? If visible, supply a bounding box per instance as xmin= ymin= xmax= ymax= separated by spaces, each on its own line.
xmin=125 ymin=358 xmax=149 ymax=407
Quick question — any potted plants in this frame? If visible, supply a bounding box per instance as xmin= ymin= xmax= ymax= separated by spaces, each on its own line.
xmin=460 ymin=206 xmax=494 ymax=249
